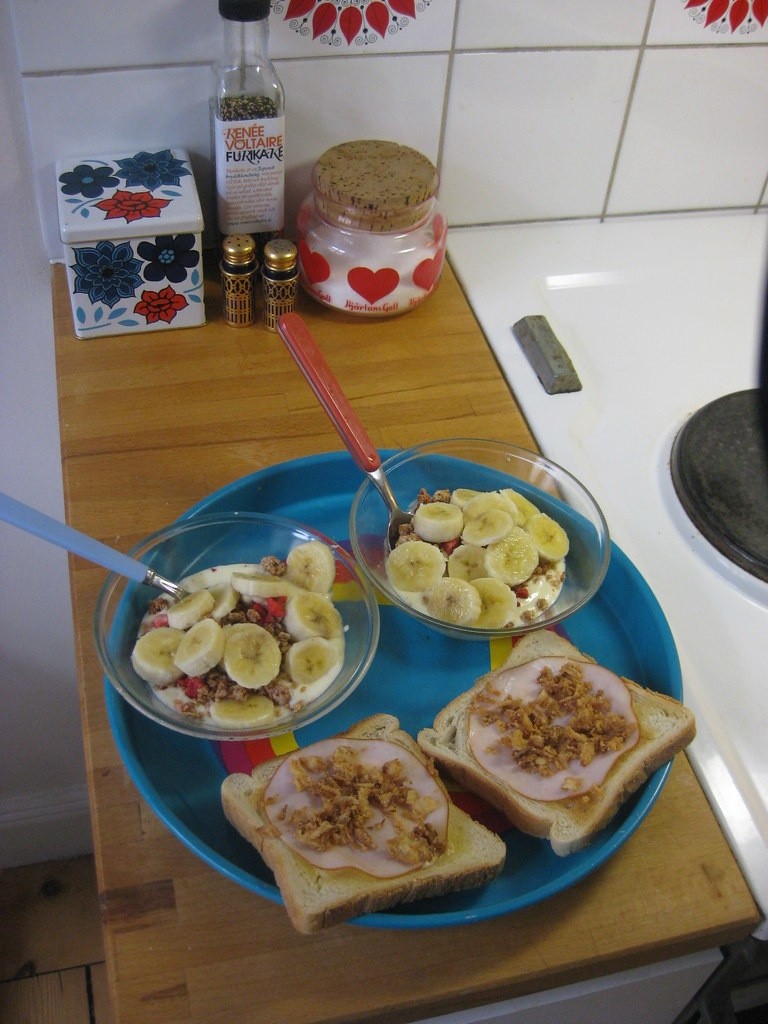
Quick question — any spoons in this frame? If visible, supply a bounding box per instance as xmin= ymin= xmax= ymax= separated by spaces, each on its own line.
xmin=279 ymin=313 xmax=414 ymax=553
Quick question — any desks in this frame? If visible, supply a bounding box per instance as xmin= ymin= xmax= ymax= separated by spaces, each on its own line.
xmin=51 ymin=239 xmax=763 ymax=1024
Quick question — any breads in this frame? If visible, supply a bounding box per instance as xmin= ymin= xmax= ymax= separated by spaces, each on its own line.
xmin=415 ymin=625 xmax=699 ymax=856
xmin=220 ymin=711 xmax=508 ymax=935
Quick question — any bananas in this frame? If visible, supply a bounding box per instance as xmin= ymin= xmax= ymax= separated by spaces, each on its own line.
xmin=384 ymin=487 xmax=570 ymax=631
xmin=131 ymin=540 xmax=343 ymax=730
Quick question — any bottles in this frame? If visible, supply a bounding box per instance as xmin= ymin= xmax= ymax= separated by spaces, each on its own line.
xmin=262 ymin=237 xmax=297 ymax=334
xmin=218 ymin=233 xmax=257 ymax=328
xmin=299 ymin=143 xmax=447 ymax=318
xmin=209 ymin=1 xmax=287 ymax=252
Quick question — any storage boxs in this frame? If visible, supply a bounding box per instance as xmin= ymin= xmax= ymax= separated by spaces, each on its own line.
xmin=58 ymin=149 xmax=211 ymax=341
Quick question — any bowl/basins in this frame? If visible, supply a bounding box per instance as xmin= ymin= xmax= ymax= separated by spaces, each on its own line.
xmin=93 ymin=511 xmax=382 ymax=741
xmin=347 ymin=437 xmax=611 ymax=639
